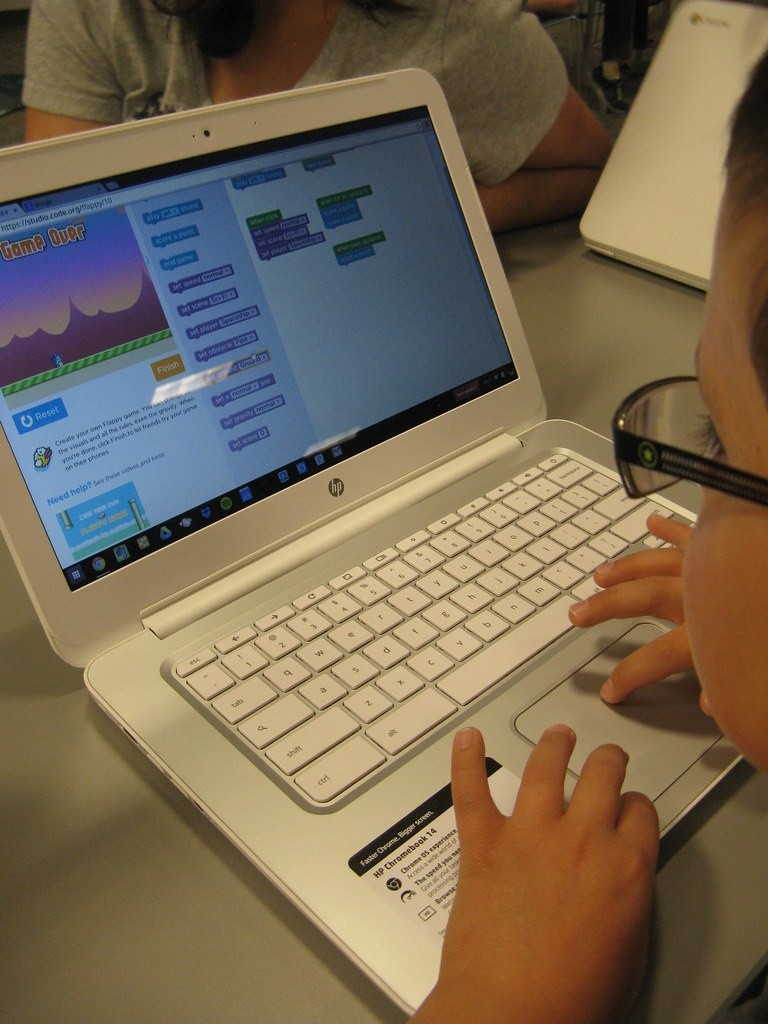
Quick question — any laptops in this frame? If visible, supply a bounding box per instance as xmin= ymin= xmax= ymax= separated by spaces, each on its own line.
xmin=578 ymin=0 xmax=768 ymax=296
xmin=1 ymin=65 xmax=754 ymax=1023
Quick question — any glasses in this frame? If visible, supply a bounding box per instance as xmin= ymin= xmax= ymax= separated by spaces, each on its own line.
xmin=611 ymin=377 xmax=768 ymax=506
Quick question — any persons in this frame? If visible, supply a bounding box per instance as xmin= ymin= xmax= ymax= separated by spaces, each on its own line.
xmin=407 ymin=50 xmax=768 ymax=1024
xmin=20 ymin=0 xmax=660 ymax=235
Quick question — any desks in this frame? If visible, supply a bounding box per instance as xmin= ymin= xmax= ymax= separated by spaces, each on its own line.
xmin=0 ymin=214 xmax=767 ymax=1024
